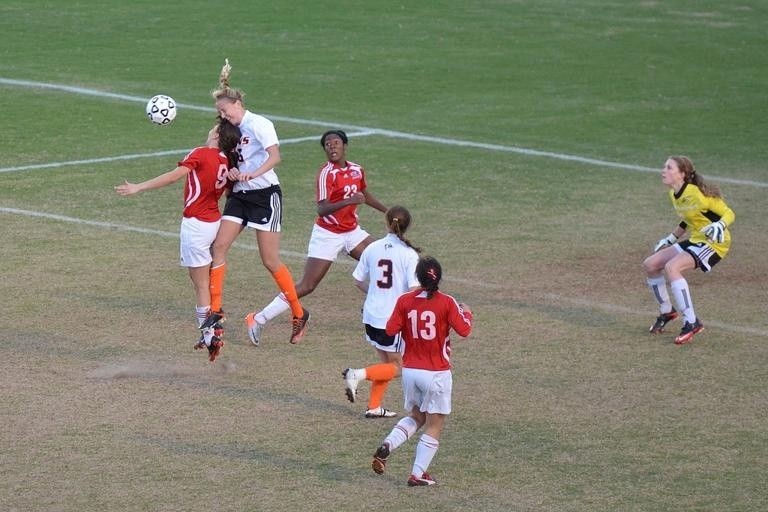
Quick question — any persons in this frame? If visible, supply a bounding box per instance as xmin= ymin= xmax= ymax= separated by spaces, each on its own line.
xmin=199 ymin=57 xmax=311 ymax=345
xmin=113 ymin=116 xmax=243 ymax=363
xmin=246 ymin=131 xmax=390 ymax=346
xmin=341 ymin=206 xmax=421 ymax=419
xmin=371 ymin=256 xmax=474 ymax=487
xmin=644 ymin=156 xmax=735 ymax=345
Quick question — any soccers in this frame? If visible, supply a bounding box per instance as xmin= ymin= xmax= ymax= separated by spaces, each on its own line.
xmin=146 ymin=94 xmax=178 ymax=126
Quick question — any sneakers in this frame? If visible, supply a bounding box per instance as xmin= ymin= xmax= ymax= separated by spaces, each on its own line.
xmin=198 ymin=310 xmax=226 ymax=329
xmin=649 ymin=308 xmax=678 ymax=335
xmin=372 ymin=442 xmax=390 ymax=477
xmin=290 ymin=309 xmax=312 ymax=343
xmin=204 ymin=336 xmax=223 ymax=363
xmin=364 ymin=406 xmax=400 ymax=420
xmin=194 ymin=321 xmax=224 ymax=350
xmin=342 ymin=367 xmax=358 ymax=406
xmin=244 ymin=311 xmax=265 ymax=349
xmin=674 ymin=319 xmax=705 ymax=344
xmin=406 ymin=474 xmax=438 ymax=487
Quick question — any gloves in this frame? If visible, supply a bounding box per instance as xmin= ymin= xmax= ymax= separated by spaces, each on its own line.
xmin=652 ymin=233 xmax=677 ymax=255
xmin=702 ymin=222 xmax=726 ymax=246
